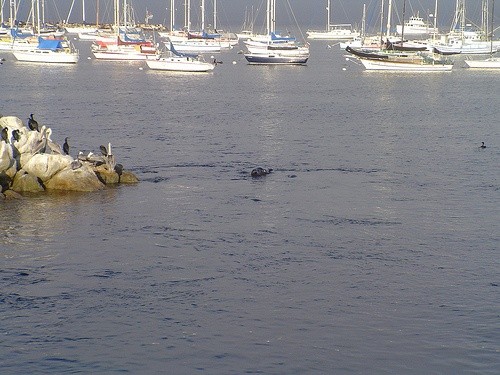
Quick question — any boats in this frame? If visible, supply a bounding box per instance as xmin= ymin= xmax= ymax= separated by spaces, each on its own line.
xmin=145 ymin=54 xmax=218 ymax=72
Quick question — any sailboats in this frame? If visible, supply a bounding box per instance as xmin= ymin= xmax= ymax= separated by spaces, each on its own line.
xmin=0 ymin=0 xmax=500 ymax=73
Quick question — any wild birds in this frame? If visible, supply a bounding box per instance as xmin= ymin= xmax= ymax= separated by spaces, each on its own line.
xmin=63 ymin=137 xmax=70 ymax=155
xmin=12 ymin=129 xmax=21 ymax=142
xmin=32 ymin=125 xmax=61 ymax=156
xmin=27 ymin=113 xmax=39 ymax=132
xmin=77 ymin=143 xmax=112 ymax=166
xmin=1 ymin=127 xmax=8 ymax=144
